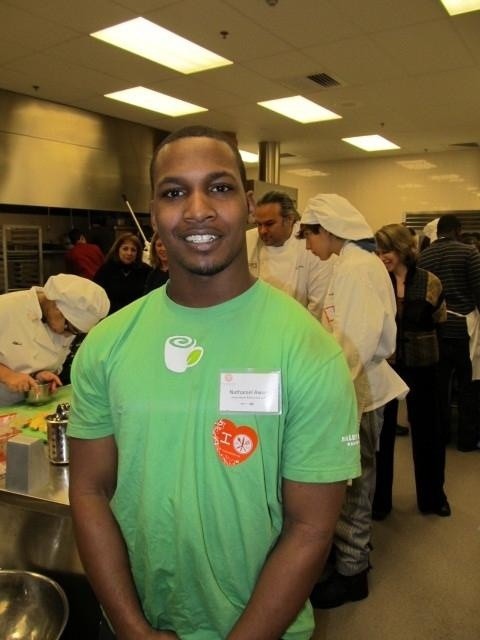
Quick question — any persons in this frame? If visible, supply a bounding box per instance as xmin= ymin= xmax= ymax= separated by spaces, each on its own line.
xmin=244 ymin=189 xmax=408 ymax=612
xmin=65 ymin=125 xmax=362 ymax=640
xmin=1 ymin=228 xmax=171 ymax=407
xmin=369 ymin=215 xmax=480 ymax=521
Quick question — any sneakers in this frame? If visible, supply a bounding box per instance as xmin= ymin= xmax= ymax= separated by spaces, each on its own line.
xmin=311 ymin=572 xmax=370 ymax=609
xmin=419 ymin=502 xmax=451 ymax=517
xmin=372 ymin=503 xmax=392 ymax=521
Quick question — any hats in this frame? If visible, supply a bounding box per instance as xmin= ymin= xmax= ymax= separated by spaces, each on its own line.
xmin=299 ymin=194 xmax=374 ymax=243
xmin=44 ymin=275 xmax=111 ymax=335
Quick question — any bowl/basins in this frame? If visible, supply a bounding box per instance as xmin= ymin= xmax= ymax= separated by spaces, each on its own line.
xmin=0 ymin=569 xmax=70 ymax=640
xmin=24 ymin=385 xmax=50 ymax=406
